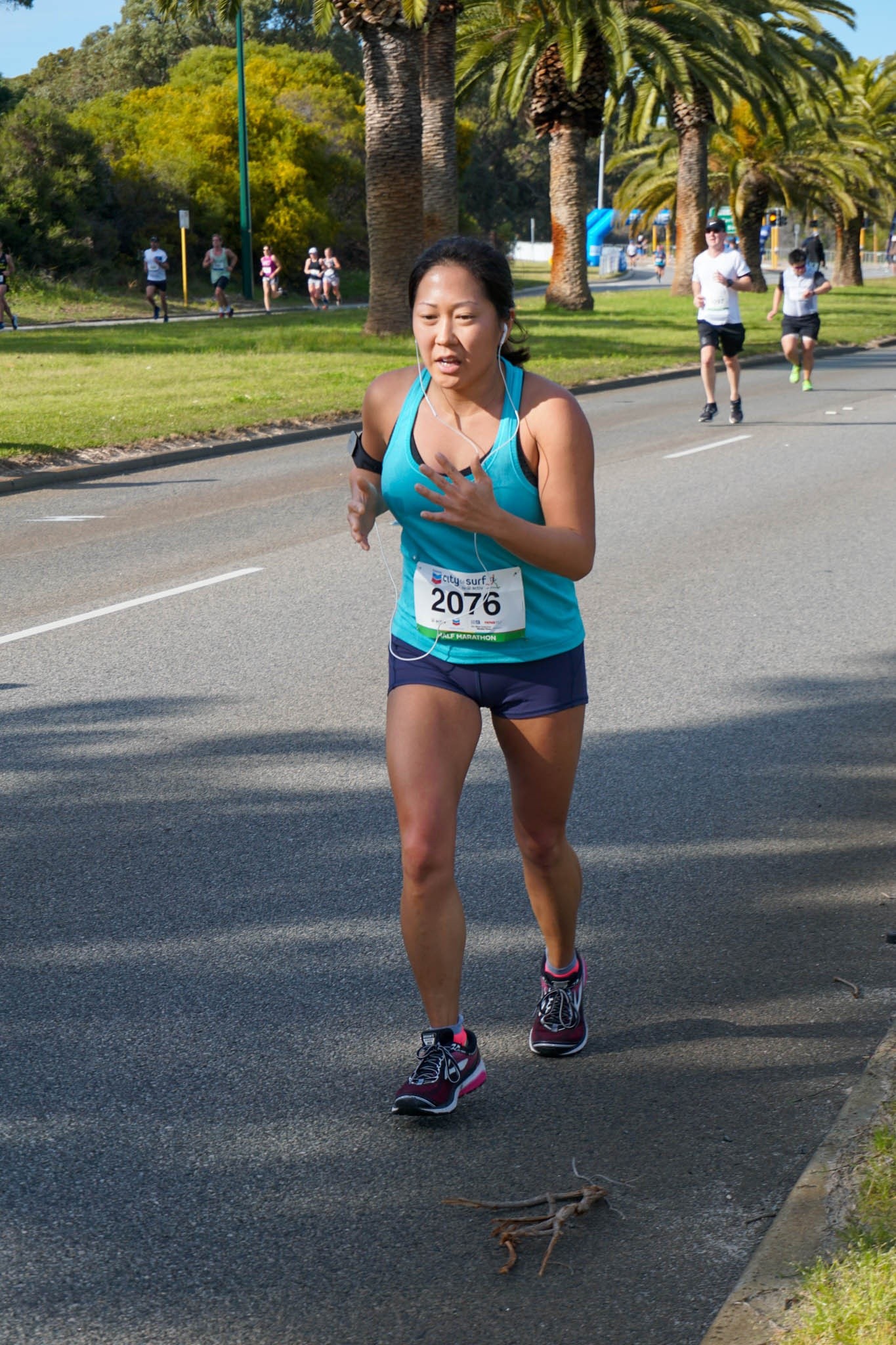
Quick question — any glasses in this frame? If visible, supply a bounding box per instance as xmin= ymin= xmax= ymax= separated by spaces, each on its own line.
xmin=705 ymin=229 xmax=720 ymax=233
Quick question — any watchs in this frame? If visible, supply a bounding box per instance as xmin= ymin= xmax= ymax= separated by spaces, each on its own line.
xmin=727 ymin=277 xmax=734 ymax=288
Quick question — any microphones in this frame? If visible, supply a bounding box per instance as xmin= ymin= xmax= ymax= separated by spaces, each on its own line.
xmin=499 ymin=322 xmax=509 ymax=347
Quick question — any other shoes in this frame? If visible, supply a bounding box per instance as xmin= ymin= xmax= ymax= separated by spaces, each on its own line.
xmin=219 ymin=312 xmax=224 ymax=318
xmin=698 ymin=404 xmax=719 ymax=424
xmin=11 ymin=315 xmax=18 ymax=330
xmin=153 ymin=307 xmax=160 ymax=319
xmin=729 ymin=394 xmax=743 ymax=424
xmin=163 ymin=316 xmax=168 ymax=323
xmin=0 ymin=324 xmax=5 ymax=331
xmin=228 ymin=309 xmax=234 ymax=317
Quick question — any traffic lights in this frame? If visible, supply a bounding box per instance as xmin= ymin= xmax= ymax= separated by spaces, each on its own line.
xmin=817 ymin=217 xmax=825 ymax=228
xmin=778 ymin=210 xmax=788 ymax=227
xmin=809 ymin=220 xmax=818 ymax=227
xmin=768 ymin=209 xmax=778 ymax=227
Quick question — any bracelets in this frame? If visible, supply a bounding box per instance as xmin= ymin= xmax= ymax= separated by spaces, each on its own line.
xmin=812 ymin=290 xmax=815 ymax=296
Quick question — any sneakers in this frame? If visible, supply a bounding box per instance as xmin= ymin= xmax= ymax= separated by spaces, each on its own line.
xmin=530 ymin=951 xmax=589 ymax=1057
xmin=802 ymin=380 xmax=813 ymax=392
xmin=391 ymin=1028 xmax=488 ymax=1116
xmin=789 ymin=363 xmax=801 ymax=384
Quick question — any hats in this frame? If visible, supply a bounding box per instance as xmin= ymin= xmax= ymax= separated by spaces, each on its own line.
xmin=308 ymin=247 xmax=318 ymax=254
xmin=704 ymin=223 xmax=725 ymax=230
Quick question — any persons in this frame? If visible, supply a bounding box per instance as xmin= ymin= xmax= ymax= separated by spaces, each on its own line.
xmin=651 ymin=244 xmax=667 ymax=283
xmin=344 ymin=233 xmax=600 ymax=1117
xmin=626 ymin=233 xmax=649 ymax=268
xmin=304 ymin=246 xmax=341 ymax=311
xmin=692 ymin=218 xmax=754 ymax=426
xmin=202 ymin=234 xmax=238 ymax=319
xmin=802 ymin=231 xmax=826 ymax=279
xmin=0 ymin=241 xmax=18 ymax=330
xmin=144 ymin=236 xmax=170 ymax=323
xmin=260 ymin=243 xmax=288 ymax=315
xmin=767 ymin=249 xmax=833 ymax=391
